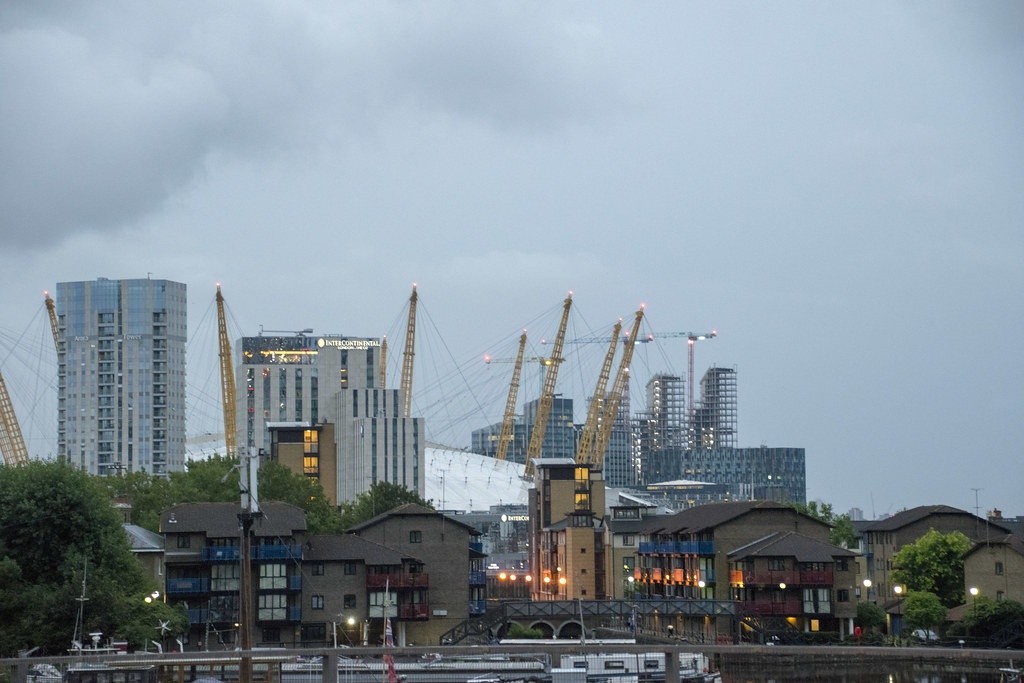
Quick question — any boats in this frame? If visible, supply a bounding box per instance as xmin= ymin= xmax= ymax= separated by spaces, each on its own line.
xmin=26 ymin=662 xmax=62 ymax=683
xmin=61 ymin=556 xmax=156 ymax=683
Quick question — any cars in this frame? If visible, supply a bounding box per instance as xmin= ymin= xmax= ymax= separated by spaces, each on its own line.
xmin=911 ymin=629 xmax=940 ymax=644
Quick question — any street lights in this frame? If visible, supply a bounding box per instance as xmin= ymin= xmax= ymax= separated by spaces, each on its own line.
xmin=894 ymin=586 xmax=902 ymax=646
xmin=544 ymin=577 xmax=550 ymax=600
xmin=629 ymin=575 xmax=634 ymax=597
xmin=970 ymin=587 xmax=979 ymax=617
xmin=864 ymin=579 xmax=871 ymax=601
xmin=560 ymin=577 xmax=567 ymax=600
xmin=698 ymin=581 xmax=705 ymax=599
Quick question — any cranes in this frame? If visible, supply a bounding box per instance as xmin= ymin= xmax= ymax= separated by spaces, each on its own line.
xmin=542 ymin=330 xmax=716 ymax=430
